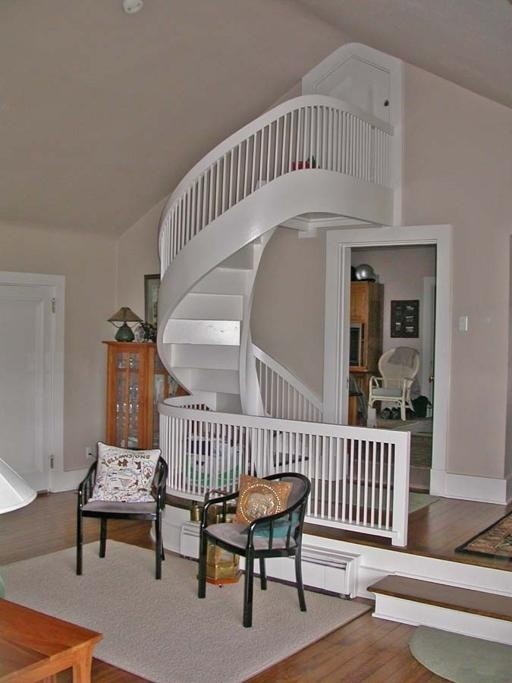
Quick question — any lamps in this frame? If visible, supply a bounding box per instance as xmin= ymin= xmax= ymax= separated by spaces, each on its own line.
xmin=0 ymin=459 xmax=37 ymax=596
xmin=107 ymin=307 xmax=143 ymax=342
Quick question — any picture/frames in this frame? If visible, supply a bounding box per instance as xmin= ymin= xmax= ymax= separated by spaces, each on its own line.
xmin=144 ymin=274 xmax=161 ymax=328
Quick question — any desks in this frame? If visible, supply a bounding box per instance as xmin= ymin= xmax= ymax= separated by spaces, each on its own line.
xmin=0 ymin=597 xmax=103 ymax=683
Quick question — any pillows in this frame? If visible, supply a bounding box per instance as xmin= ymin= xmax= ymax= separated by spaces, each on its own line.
xmin=93 ymin=443 xmax=161 ymax=503
xmin=234 ymin=474 xmax=300 ymax=538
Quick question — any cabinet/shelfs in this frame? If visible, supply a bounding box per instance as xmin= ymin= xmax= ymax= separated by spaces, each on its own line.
xmin=350 ymin=281 xmax=383 ymax=401
xmin=102 ymin=340 xmax=189 ymax=449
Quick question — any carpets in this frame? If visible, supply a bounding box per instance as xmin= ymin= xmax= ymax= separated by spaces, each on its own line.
xmin=0 ymin=537 xmax=372 ymax=683
xmin=454 ymin=509 xmax=512 ymax=561
xmin=310 ymin=480 xmax=441 ymax=515
xmin=410 ymin=626 xmax=512 ymax=683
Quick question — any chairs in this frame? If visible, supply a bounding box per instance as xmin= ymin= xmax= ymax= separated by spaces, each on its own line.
xmin=198 ymin=472 xmax=312 ymax=627
xmin=77 ymin=453 xmax=168 ymax=579
xmin=368 ymin=346 xmax=421 ymax=421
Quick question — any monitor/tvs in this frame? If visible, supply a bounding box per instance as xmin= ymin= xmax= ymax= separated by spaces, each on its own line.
xmin=350 ymin=322 xmax=363 ymax=366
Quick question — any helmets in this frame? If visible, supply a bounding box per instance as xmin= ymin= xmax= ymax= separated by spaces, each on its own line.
xmin=356 ymin=264 xmax=376 ymax=280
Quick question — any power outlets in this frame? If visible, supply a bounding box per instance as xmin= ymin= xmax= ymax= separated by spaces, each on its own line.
xmin=86 ymin=447 xmax=92 ymax=458
